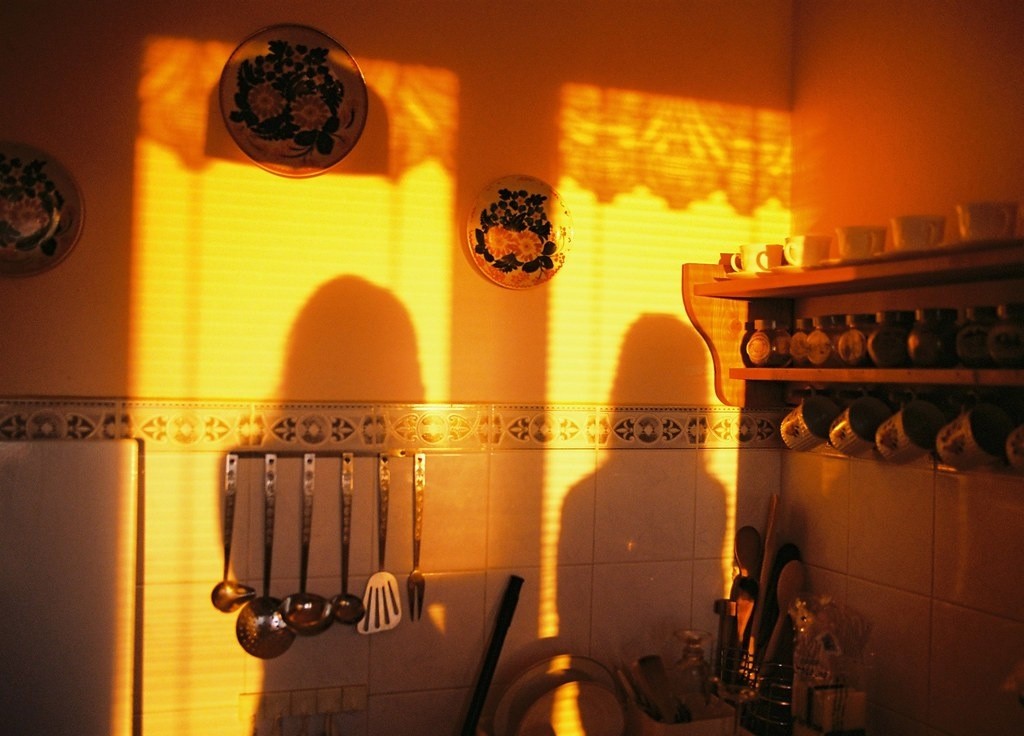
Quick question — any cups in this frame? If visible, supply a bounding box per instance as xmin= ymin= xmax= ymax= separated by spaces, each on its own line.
xmin=1005 ymin=422 xmax=1024 ymax=474
xmin=828 ymin=395 xmax=893 ymax=458
xmin=952 ymin=201 xmax=1019 ymax=242
xmin=779 ymin=384 xmax=841 ymax=453
xmin=729 ymin=243 xmax=783 ymax=274
xmin=874 ymin=388 xmax=947 ymax=467
xmin=783 ymin=234 xmax=833 ymax=265
xmin=935 ymin=402 xmax=1014 ymax=471
xmin=638 ymin=693 xmax=735 ymax=735
xmin=888 ymin=214 xmax=946 ymax=249
xmin=833 ymin=225 xmax=887 ymax=258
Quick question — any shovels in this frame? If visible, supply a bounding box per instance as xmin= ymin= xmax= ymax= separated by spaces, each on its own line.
xmin=734 ymin=524 xmax=806 ymax=691
xmin=357 ymin=454 xmax=403 ymax=634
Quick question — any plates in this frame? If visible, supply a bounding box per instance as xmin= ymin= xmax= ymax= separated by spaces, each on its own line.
xmin=771 ymin=263 xmax=826 ymax=274
xmin=515 ymin=680 xmax=627 ymax=736
xmin=725 ymin=269 xmax=774 ymax=279
xmin=218 ymin=23 xmax=368 ymax=179
xmin=465 ymin=173 xmax=576 ymax=291
xmin=819 ymin=242 xmax=983 ymax=267
xmin=0 ymin=140 xmax=85 ymax=274
xmin=493 ymin=653 xmax=618 ymax=736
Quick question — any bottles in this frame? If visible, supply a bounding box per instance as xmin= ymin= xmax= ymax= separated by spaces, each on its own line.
xmin=669 ymin=628 xmax=725 ymax=718
xmin=746 ymin=303 xmax=1024 ymax=368
